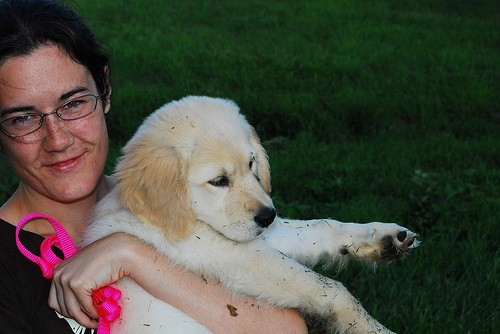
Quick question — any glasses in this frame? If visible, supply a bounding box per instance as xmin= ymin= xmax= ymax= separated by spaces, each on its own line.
xmin=0 ymin=81 xmax=106 ymax=138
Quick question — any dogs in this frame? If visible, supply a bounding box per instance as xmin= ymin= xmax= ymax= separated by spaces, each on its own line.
xmin=76 ymin=95 xmax=423 ymax=334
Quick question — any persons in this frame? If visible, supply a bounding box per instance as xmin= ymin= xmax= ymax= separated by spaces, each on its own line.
xmin=0 ymin=0 xmax=307 ymax=334
